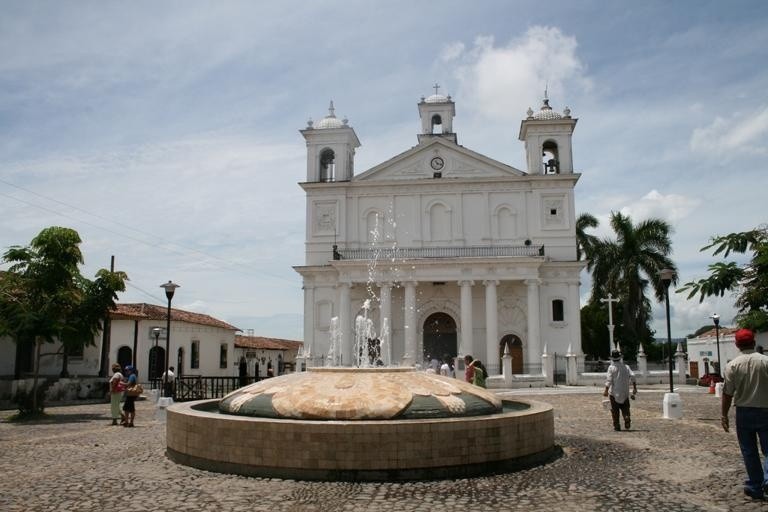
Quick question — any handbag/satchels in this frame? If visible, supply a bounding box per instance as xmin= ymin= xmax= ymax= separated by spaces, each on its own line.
xmin=111 ymin=381 xmax=126 ymax=393
xmin=126 ymin=383 xmax=143 ymax=397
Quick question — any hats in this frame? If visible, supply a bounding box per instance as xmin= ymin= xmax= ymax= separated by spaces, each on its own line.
xmin=734 ymin=328 xmax=755 ymax=341
xmin=111 ymin=363 xmax=134 ymax=371
xmin=608 ymin=349 xmax=623 ymax=360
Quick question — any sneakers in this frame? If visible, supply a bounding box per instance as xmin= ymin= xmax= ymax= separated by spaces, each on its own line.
xmin=614 ymin=416 xmax=630 ymax=431
xmin=743 ymin=484 xmax=762 ymax=500
xmin=110 ymin=417 xmax=134 ymax=427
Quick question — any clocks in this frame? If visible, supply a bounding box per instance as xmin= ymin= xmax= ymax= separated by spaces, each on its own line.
xmin=431 ymin=156 xmax=444 ymax=171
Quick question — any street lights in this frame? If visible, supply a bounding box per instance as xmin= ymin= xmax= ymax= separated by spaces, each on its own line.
xmin=157 ymin=281 xmax=180 ymax=410
xmin=662 ymin=337 xmax=666 ymax=370
xmin=153 ymin=327 xmax=163 ymax=389
xmin=710 ymin=313 xmax=726 ymax=398
xmin=654 ymin=267 xmax=683 ymax=419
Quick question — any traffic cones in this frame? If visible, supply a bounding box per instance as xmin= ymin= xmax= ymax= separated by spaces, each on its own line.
xmin=708 ymin=379 xmax=714 ymax=394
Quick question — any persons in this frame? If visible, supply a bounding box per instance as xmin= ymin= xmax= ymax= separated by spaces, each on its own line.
xmin=162 ymin=367 xmax=175 ymax=397
xmin=464 ymin=354 xmax=474 ymax=384
xmin=119 ymin=366 xmax=136 ymax=427
xmin=603 ymin=350 xmax=637 ymax=430
xmin=720 ymin=329 xmax=767 ymax=499
xmin=441 ymin=354 xmax=455 ymax=379
xmin=756 ymin=345 xmax=763 ymax=354
xmin=109 ymin=363 xmax=125 ymax=425
xmin=469 ymin=359 xmax=488 ymax=389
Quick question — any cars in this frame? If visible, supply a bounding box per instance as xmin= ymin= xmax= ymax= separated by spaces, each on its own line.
xmin=699 ymin=372 xmax=724 ymax=386
xmin=685 ymin=374 xmax=697 ymax=385
